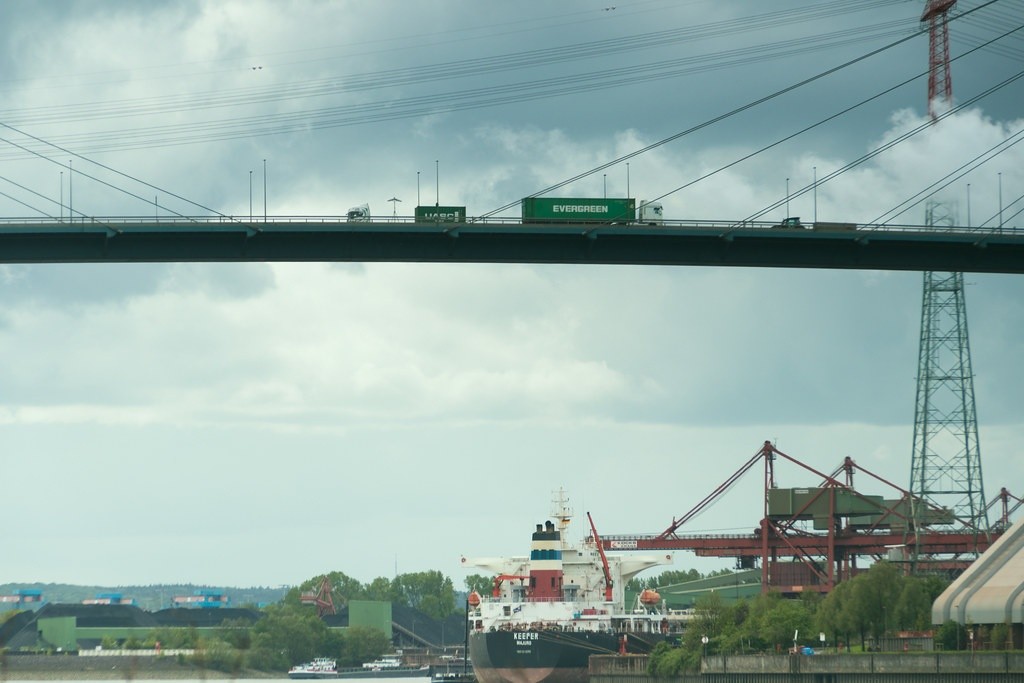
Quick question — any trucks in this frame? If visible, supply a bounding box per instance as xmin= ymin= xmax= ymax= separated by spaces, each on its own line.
xmin=415 ymin=205 xmax=473 ymax=225
xmin=771 ymin=217 xmax=857 ymax=233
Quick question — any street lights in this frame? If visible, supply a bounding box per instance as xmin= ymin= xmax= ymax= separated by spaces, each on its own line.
xmin=60 ymin=172 xmax=63 ymax=224
xmin=812 ymin=167 xmax=818 ymax=223
xmin=249 ymin=170 xmax=252 ymax=222
xmin=819 ymin=629 xmax=826 ymax=657
xmin=998 ymin=172 xmax=1003 ymax=235
xmin=603 ymin=174 xmax=607 ymax=199
xmin=417 ymin=171 xmax=420 ymax=207
xmin=626 ymin=162 xmax=630 ymax=227
xmin=701 ymin=634 xmax=710 ymax=661
xmin=263 ymin=159 xmax=267 ymax=222
xmin=69 ymin=159 xmax=73 ymax=224
xmin=435 ymin=160 xmax=440 ymax=223
xmin=786 ymin=178 xmax=789 ymax=220
xmin=967 ymin=183 xmax=971 ymax=233
xmin=967 ymin=629 xmax=975 ymax=653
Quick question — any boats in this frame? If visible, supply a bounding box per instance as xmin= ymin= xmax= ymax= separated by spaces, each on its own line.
xmin=460 ymin=483 xmax=709 ymax=683
xmin=287 ymin=650 xmax=428 ymax=683
xmin=430 ymin=646 xmax=479 ymax=683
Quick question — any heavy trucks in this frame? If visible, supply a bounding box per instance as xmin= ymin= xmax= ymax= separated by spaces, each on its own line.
xmin=521 ymin=196 xmax=665 ymax=228
